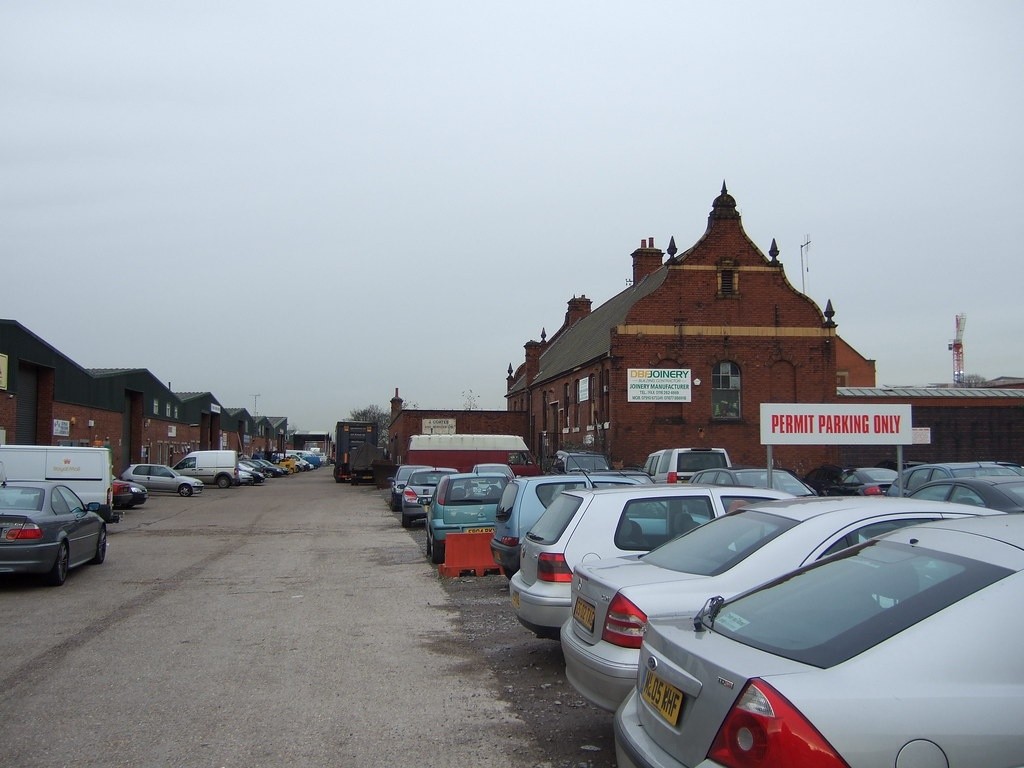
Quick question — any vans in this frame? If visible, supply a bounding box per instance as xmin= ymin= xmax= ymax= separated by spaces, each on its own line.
xmin=547 ymin=449 xmax=609 ymax=474
xmin=0 ymin=445 xmax=123 ymax=528
xmin=160 ymin=449 xmax=240 ymax=489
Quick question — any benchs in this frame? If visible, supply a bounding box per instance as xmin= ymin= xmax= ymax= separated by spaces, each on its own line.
xmin=619 ymin=517 xmax=652 ymax=547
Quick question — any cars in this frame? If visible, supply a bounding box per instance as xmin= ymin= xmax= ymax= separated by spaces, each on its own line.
xmin=491 ymin=447 xmax=1024 ymax=768
xmin=386 ymin=464 xmax=439 ymax=512
xmin=415 ymin=472 xmax=516 ymax=564
xmin=119 ymin=463 xmax=204 ymax=498
xmin=111 ymin=475 xmax=148 ymax=510
xmin=469 ymin=463 xmax=522 ymax=498
xmin=397 ymin=466 xmax=473 ymax=527
xmin=0 ymin=478 xmax=109 ymax=588
xmin=238 ymin=449 xmax=329 ymax=486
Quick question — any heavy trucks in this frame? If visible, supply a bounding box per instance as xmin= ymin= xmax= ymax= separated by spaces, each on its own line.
xmin=331 ymin=420 xmax=379 ymax=484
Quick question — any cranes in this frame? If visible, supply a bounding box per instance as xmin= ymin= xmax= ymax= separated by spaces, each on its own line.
xmin=948 ymin=312 xmax=966 ymax=387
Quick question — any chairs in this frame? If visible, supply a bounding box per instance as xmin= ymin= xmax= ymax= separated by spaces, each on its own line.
xmin=451 ymin=487 xmax=466 ymax=499
xmin=873 ymin=564 xmax=921 ymax=608
xmin=486 ymin=485 xmax=502 ymax=497
xmin=674 ymin=512 xmax=693 ymax=539
xmin=415 ymin=475 xmax=428 ymax=484
